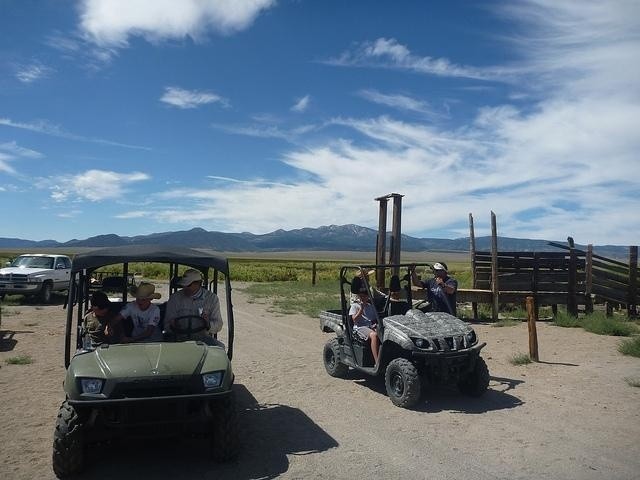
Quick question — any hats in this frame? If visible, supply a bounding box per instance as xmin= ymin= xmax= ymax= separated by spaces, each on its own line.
xmin=356 ymin=268 xmax=375 ymax=278
xmin=91 ymin=292 xmax=111 ymax=309
xmin=176 ymin=269 xmax=201 ymax=286
xmin=430 ymin=263 xmax=448 ymax=273
xmin=129 ymin=282 xmax=161 ymax=300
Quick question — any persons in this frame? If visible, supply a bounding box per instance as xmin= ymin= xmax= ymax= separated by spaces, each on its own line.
xmin=410 ymin=262 xmax=457 ymax=316
xmin=349 ymin=268 xmax=400 ymax=366
xmin=82 ymin=291 xmax=127 ymax=344
xmin=163 ymin=269 xmax=223 ymax=342
xmin=105 ymin=283 xmax=160 ymax=341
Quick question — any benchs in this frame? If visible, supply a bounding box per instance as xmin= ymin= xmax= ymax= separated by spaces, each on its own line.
xmin=346 ymin=301 xmax=407 ymax=346
xmin=112 ymin=301 xmax=165 ymax=336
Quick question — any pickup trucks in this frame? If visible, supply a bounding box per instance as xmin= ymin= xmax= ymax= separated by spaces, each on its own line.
xmin=0 ymin=254 xmax=86 ymax=304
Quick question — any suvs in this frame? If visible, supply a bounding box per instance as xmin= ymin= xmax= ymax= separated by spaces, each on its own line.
xmin=319 ymin=264 xmax=490 ymax=408
xmin=52 ymin=247 xmax=237 ymax=478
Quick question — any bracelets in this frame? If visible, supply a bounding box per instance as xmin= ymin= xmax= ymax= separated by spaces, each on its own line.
xmin=442 ymin=283 xmax=447 ymax=289
